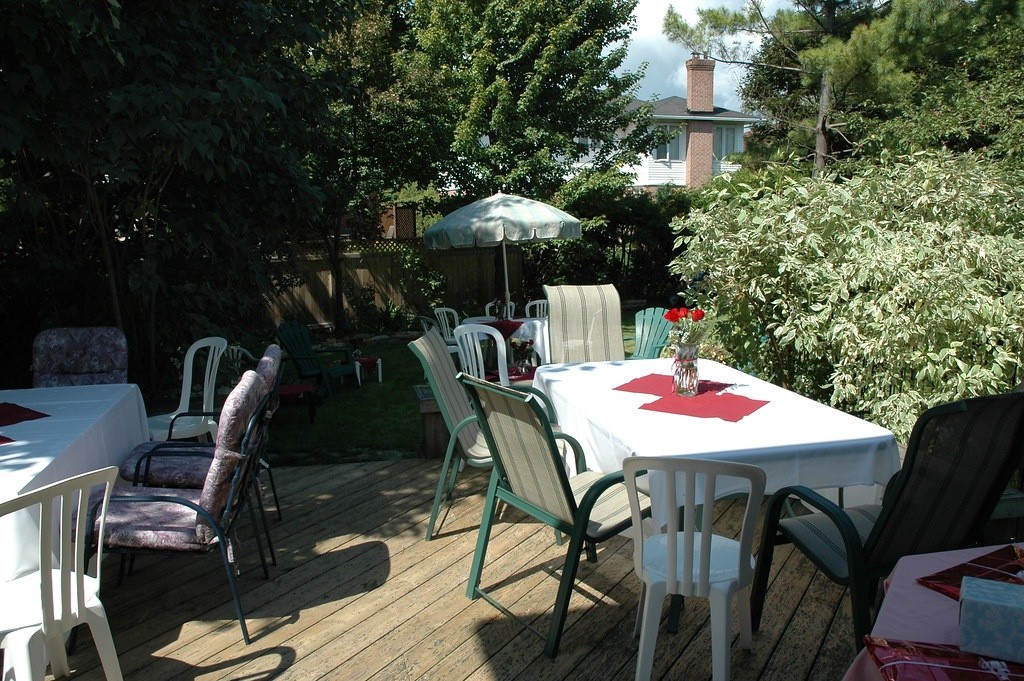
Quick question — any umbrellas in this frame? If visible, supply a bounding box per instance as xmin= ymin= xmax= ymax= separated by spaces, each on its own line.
xmin=424 ymin=190 xmax=580 ymax=321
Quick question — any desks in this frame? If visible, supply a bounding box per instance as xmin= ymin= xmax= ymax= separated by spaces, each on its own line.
xmin=279 ymin=384 xmax=316 ymax=424
xmin=531 ymin=357 xmax=902 ymax=635
xmin=841 ymin=543 xmax=1024 ymax=681
xmin=0 ymin=384 xmax=152 ymax=581
xmin=333 ymin=356 xmax=382 ymax=386
xmin=461 ymin=317 xmax=551 ymax=366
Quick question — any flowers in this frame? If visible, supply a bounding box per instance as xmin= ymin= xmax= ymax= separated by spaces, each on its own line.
xmin=510 ymin=339 xmax=534 ymax=360
xmin=349 ymin=336 xmax=364 ymax=348
xmin=665 ymin=307 xmax=705 ymax=342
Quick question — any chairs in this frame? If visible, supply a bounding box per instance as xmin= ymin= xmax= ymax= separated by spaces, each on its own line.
xmin=452 ymin=324 xmax=510 ymax=409
xmin=421 ymin=316 xmax=466 ymax=380
xmin=485 ymin=301 xmax=515 ymax=347
xmin=33 ymin=327 xmax=128 ymax=388
xmin=455 ymin=371 xmax=686 ymax=659
xmin=216 ymin=346 xmax=262 ymax=394
xmin=542 ymin=283 xmax=626 ymax=364
xmin=59 ymin=370 xmax=271 ymax=655
xmin=147 ymin=337 xmax=227 ymax=444
xmin=0 ymin=465 xmax=136 ymax=681
xmin=276 ymin=320 xmax=360 ymax=399
xmin=751 ymin=389 xmax=1024 ymax=656
xmin=525 ymin=300 xmax=548 ymax=318
xmin=117 ymin=344 xmax=284 ymax=575
xmin=622 ymin=457 xmax=767 ymax=681
xmin=623 ymin=307 xmax=676 ymax=360
xmin=434 ymin=308 xmax=489 ymax=370
xmin=408 ymin=325 xmax=568 ymax=546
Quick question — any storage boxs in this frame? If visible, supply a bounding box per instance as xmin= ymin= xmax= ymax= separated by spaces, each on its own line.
xmin=959 ymin=576 xmax=1024 ymax=664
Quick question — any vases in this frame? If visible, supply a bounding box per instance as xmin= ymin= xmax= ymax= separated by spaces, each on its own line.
xmin=673 ymin=342 xmax=701 ymax=397
xmin=515 ymin=360 xmax=531 ymax=374
xmin=352 ymin=349 xmax=362 ymax=361
